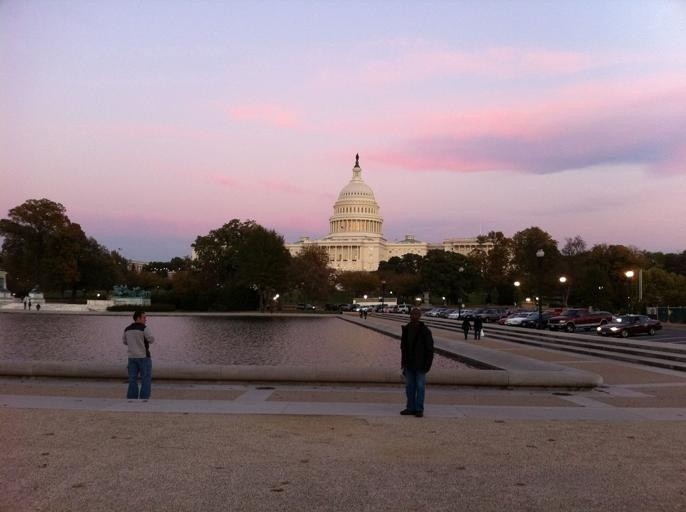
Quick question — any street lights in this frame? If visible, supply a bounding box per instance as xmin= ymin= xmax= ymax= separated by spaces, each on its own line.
xmin=514 ymin=281 xmax=520 ymax=307
xmin=559 ymin=276 xmax=567 ymax=310
xmin=624 ymin=266 xmax=634 ymax=314
xmin=536 ymin=248 xmax=545 ymax=329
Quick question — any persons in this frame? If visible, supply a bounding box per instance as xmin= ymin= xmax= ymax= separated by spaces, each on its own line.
xmin=120 ymin=309 xmax=154 ymax=399
xmin=36 ymin=303 xmax=40 ymax=311
xmin=474 ymin=317 xmax=483 ymax=340
xmin=23 ymin=300 xmax=26 ymax=310
xmin=28 ymin=299 xmax=31 ymax=310
xmin=462 ymin=317 xmax=472 ymax=340
xmin=358 ymin=303 xmax=411 ymax=320
xmin=399 ymin=306 xmax=433 ymax=418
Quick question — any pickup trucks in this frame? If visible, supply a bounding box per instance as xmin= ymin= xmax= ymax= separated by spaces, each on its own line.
xmin=547 ymin=307 xmax=611 ymax=332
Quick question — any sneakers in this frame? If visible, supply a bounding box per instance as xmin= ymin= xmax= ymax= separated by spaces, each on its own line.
xmin=400 ymin=409 xmax=423 ymax=417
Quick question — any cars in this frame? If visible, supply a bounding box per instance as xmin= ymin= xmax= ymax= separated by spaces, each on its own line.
xmin=325 ymin=304 xmax=414 ymax=312
xmin=500 ymin=308 xmax=563 ymax=328
xmin=297 ymin=304 xmax=316 ymax=311
xmin=597 ymin=313 xmax=662 ymax=337
xmin=425 ymin=306 xmax=500 ymax=323
